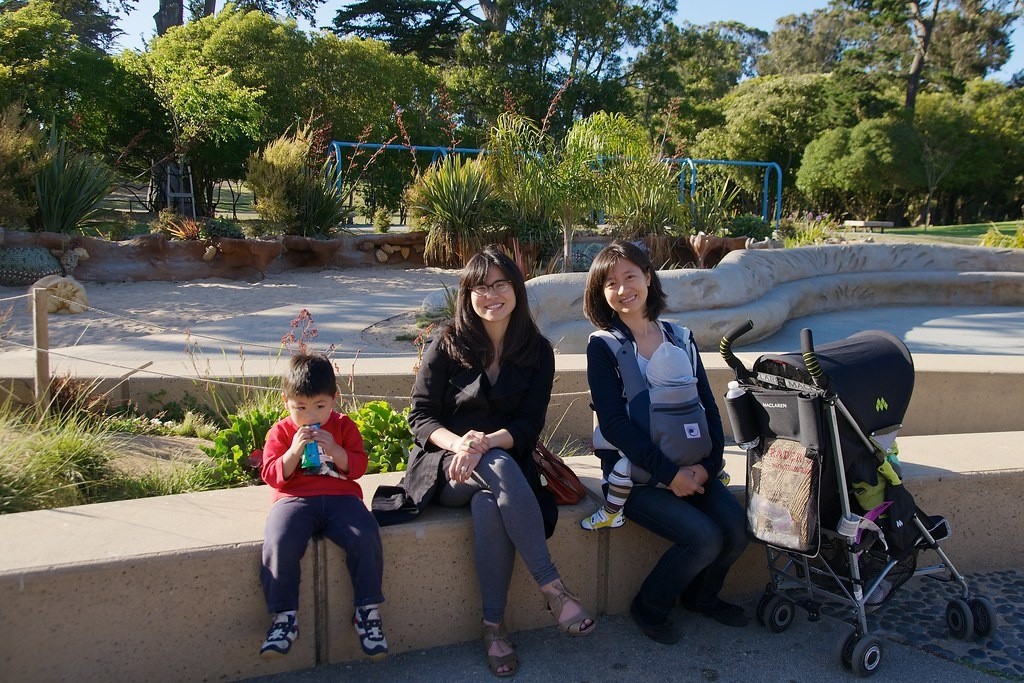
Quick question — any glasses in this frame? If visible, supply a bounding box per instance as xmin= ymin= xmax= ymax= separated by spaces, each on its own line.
xmin=467 ymin=279 xmax=512 ymax=295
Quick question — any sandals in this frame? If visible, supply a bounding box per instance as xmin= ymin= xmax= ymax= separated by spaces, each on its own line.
xmin=480 ymin=617 xmax=519 ymax=676
xmin=540 ymin=578 xmax=597 ymax=636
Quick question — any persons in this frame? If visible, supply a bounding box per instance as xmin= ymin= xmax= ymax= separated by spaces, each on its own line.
xmin=396 ymin=250 xmax=598 ymax=679
xmin=582 ymin=242 xmax=753 ymax=646
xmin=580 ymin=342 xmax=731 ymax=531
xmin=259 ymin=354 xmax=390 ymax=662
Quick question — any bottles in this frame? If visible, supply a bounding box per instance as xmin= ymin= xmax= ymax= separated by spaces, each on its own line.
xmin=725 ymin=381 xmax=760 ymax=451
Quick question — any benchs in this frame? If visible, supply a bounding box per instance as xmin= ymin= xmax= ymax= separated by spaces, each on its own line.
xmin=844 ymin=220 xmax=894 ymax=233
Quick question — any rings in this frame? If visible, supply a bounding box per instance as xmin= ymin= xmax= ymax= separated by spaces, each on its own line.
xmin=468 ymin=440 xmax=474 ymax=447
xmin=460 ymin=465 xmax=467 ymax=471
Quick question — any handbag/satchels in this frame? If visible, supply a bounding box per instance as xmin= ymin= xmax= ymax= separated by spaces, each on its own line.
xmin=532 ymin=440 xmax=585 ymax=505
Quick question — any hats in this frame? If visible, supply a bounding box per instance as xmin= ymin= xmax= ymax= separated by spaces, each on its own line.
xmin=646 ymin=341 xmax=694 ymax=388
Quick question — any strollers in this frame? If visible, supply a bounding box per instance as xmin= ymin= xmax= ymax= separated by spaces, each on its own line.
xmin=718 ymin=319 xmax=999 ymax=678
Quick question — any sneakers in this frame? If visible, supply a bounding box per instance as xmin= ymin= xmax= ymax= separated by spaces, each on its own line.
xmin=580 ymin=505 xmax=626 ymax=531
xmin=259 ymin=614 xmax=301 ymax=659
xmin=719 ymin=471 xmax=730 ymax=486
xmin=351 ymin=606 xmax=389 ymax=659
